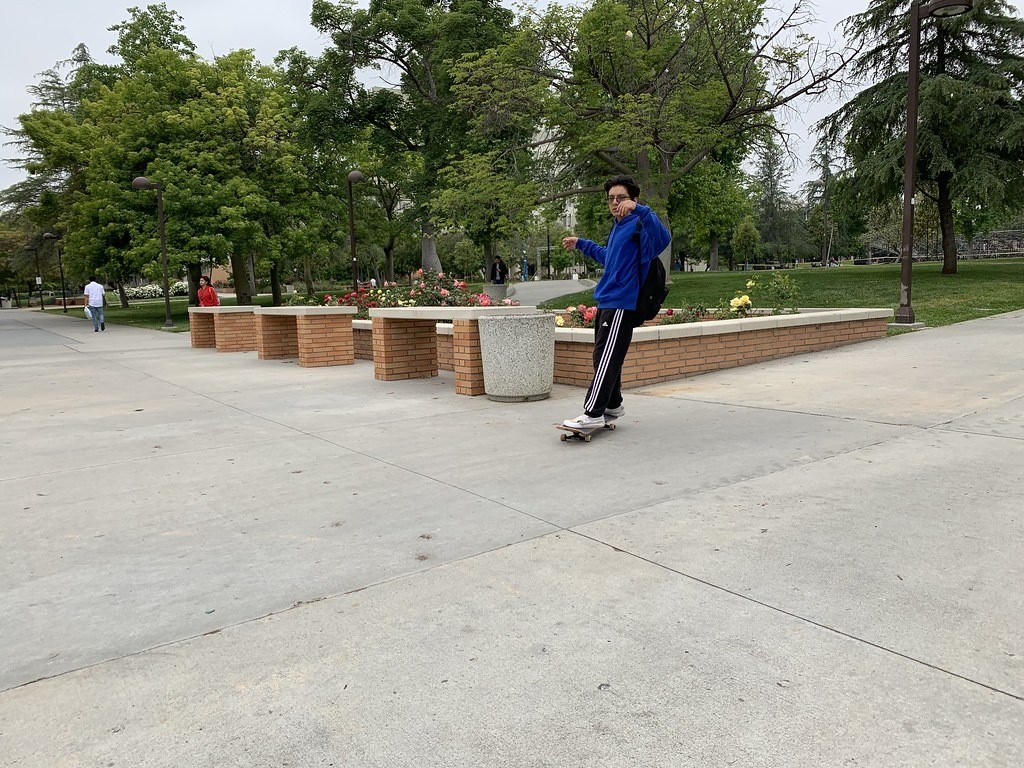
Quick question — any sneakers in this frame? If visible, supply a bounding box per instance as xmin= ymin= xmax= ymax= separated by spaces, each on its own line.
xmin=564 ymin=414 xmax=605 ymax=428
xmin=604 ymin=403 xmax=625 ymax=417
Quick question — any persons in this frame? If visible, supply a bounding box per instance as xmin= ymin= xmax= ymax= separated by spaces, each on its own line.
xmin=792 ymin=258 xmax=798 ymax=266
xmin=84 ymin=276 xmax=106 ymax=332
xmin=563 ymin=176 xmax=670 ymax=429
xmin=745 ymin=259 xmax=748 ymax=270
xmin=197 ymin=276 xmax=220 ymax=307
xmin=674 ymin=259 xmax=682 ymax=271
xmin=828 ymin=257 xmax=835 ymax=267
xmin=491 ymin=255 xmax=507 ymax=284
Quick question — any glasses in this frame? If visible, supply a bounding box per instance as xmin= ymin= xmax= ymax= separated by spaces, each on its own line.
xmin=608 ymin=196 xmax=631 ymax=203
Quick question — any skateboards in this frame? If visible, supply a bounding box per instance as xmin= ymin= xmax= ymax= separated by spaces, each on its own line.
xmin=552 ymin=413 xmax=618 ymax=442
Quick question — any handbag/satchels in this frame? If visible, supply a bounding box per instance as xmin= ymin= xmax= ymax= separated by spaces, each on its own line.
xmin=209 ymin=287 xmax=220 ymax=306
xmin=84 ymin=307 xmax=93 ymax=320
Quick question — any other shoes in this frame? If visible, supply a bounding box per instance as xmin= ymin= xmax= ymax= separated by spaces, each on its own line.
xmin=95 ymin=330 xmax=98 ymax=332
xmin=101 ymin=323 xmax=105 ymax=331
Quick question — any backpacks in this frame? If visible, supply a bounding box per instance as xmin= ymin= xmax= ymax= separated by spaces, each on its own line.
xmin=606 ymin=220 xmax=670 ymax=327
